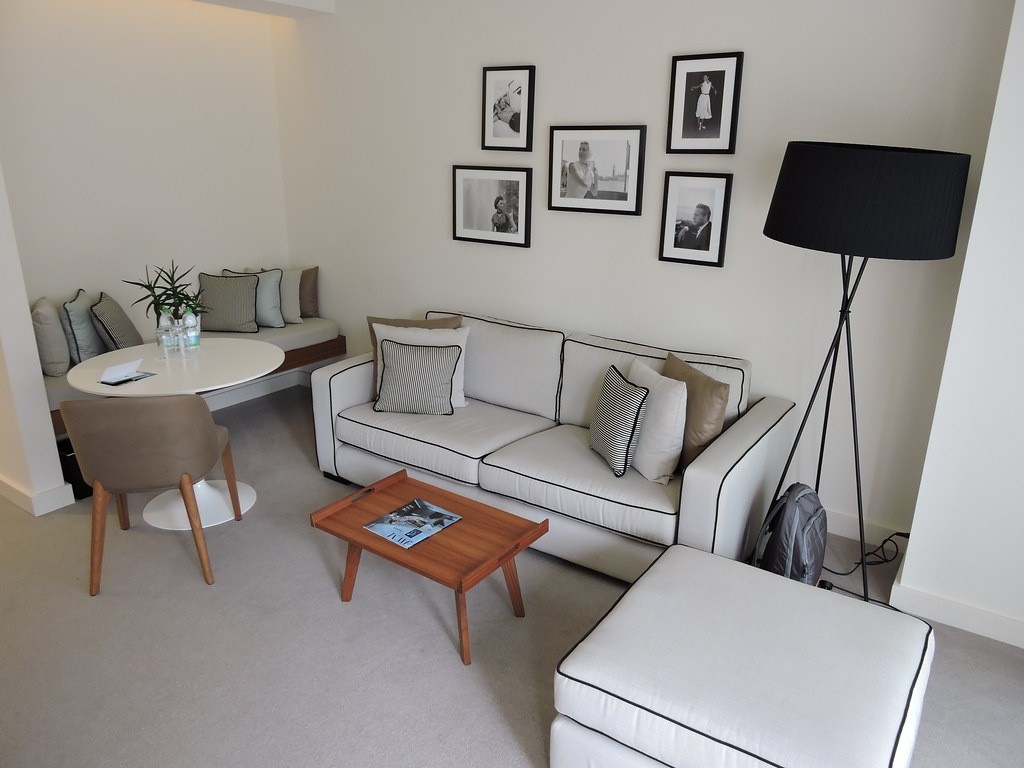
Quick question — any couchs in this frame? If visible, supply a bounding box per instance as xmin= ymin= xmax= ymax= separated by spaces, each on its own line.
xmin=310 ymin=309 xmax=796 ymax=583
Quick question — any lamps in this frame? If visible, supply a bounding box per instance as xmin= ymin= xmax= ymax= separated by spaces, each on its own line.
xmin=763 ymin=141 xmax=972 ymax=599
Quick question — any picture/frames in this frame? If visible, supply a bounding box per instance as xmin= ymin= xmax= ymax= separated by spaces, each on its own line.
xmin=549 ymin=125 xmax=648 ymax=216
xmin=666 ymin=51 xmax=744 ymax=154
xmin=659 ymin=171 xmax=733 ymax=267
xmin=481 ymin=65 xmax=535 ymax=152
xmin=452 ymin=165 xmax=533 ymax=249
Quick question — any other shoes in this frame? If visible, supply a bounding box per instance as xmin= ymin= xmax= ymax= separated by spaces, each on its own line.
xmin=699 ymin=123 xmax=702 ymax=130
xmin=702 ymin=126 xmax=705 ymax=129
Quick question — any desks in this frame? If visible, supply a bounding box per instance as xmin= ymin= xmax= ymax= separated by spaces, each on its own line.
xmin=67 ymin=338 xmax=287 ymax=529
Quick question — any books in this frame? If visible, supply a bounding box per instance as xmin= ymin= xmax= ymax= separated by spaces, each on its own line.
xmin=361 ymin=497 xmax=463 ymax=550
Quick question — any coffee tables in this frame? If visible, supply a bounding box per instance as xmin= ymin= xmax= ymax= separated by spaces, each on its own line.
xmin=310 ymin=469 xmax=549 ymax=665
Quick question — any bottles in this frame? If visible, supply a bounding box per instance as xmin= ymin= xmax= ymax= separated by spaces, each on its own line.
xmin=159 ymin=308 xmax=179 ymax=351
xmin=181 ymin=308 xmax=200 ymax=349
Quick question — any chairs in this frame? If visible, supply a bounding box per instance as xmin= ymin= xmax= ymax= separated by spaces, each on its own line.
xmin=59 ymin=395 xmax=243 ymax=596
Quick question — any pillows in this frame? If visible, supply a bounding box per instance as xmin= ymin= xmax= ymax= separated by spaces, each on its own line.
xmin=32 ymin=297 xmax=71 ymax=377
xmin=61 ymin=289 xmax=107 ymax=364
xmin=374 ymin=339 xmax=462 ymax=416
xmin=87 ymin=291 xmax=144 ymax=352
xmin=260 ymin=265 xmax=319 ymax=318
xmin=663 ymin=351 xmax=731 ymax=473
xmin=366 ymin=314 xmax=462 ymax=401
xmin=627 ymin=355 xmax=688 ymax=484
xmin=589 ymin=365 xmax=650 ymax=476
xmin=250 ymin=268 xmax=304 ymax=324
xmin=198 ymin=273 xmax=259 ymax=333
xmin=221 ymin=268 xmax=286 ymax=328
xmin=371 ymin=321 xmax=469 ymax=409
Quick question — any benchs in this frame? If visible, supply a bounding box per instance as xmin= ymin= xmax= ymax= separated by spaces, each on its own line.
xmin=45 ymin=318 xmax=347 ymax=499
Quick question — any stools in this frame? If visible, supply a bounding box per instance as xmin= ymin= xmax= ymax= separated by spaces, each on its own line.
xmin=550 ymin=542 xmax=936 ymax=768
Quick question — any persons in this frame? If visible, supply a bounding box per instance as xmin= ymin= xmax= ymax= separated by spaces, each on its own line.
xmin=675 ymin=204 xmax=712 ymax=251
xmin=390 ymin=511 xmax=430 ymax=533
xmin=690 ymin=74 xmax=717 ymax=131
xmin=562 ymin=141 xmax=598 ymax=199
xmin=490 ymin=196 xmax=517 ymax=233
xmin=493 ymin=96 xmax=520 ymax=132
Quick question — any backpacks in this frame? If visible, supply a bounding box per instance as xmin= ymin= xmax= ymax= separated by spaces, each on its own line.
xmin=745 ymin=482 xmax=827 ymax=587
